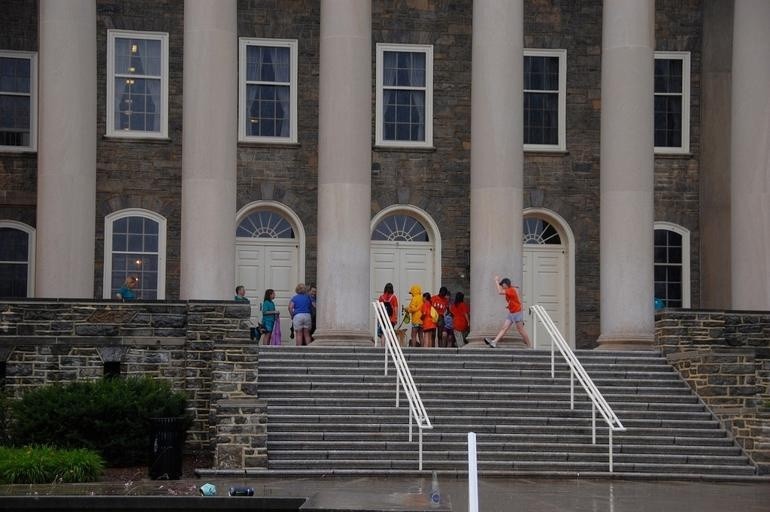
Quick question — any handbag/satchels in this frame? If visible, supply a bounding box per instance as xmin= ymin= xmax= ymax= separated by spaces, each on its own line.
xmin=443 ymin=305 xmax=453 ymax=330
xmin=257 ymin=321 xmax=267 ymax=334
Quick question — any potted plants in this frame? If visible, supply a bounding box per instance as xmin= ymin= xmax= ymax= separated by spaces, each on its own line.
xmin=144 ymin=385 xmax=192 ymax=481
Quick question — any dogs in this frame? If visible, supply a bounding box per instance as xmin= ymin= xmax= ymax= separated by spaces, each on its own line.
xmin=250 ymin=322 xmax=269 ymax=344
xmin=391 ymin=329 xmax=407 ymax=347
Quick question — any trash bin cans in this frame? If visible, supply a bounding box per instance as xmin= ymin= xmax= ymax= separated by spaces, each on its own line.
xmin=655 ymin=298 xmax=666 ymax=310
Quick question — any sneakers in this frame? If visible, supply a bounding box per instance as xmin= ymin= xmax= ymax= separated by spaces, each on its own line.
xmin=484 ymin=337 xmax=496 ymax=348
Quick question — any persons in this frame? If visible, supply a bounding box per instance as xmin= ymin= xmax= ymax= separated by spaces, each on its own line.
xmin=288 ymin=283 xmax=317 ymax=346
xmin=233 ymin=285 xmax=249 ymax=302
xmin=376 ymin=282 xmax=470 ymax=348
xmin=484 ymin=275 xmax=532 ymax=349
xmin=261 ymin=289 xmax=280 ymax=345
xmin=116 ymin=274 xmax=137 ymax=300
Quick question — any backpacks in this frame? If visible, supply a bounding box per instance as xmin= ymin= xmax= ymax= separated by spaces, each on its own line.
xmin=429 ymin=307 xmax=439 ymax=323
xmin=381 ymin=295 xmax=394 ymax=316
